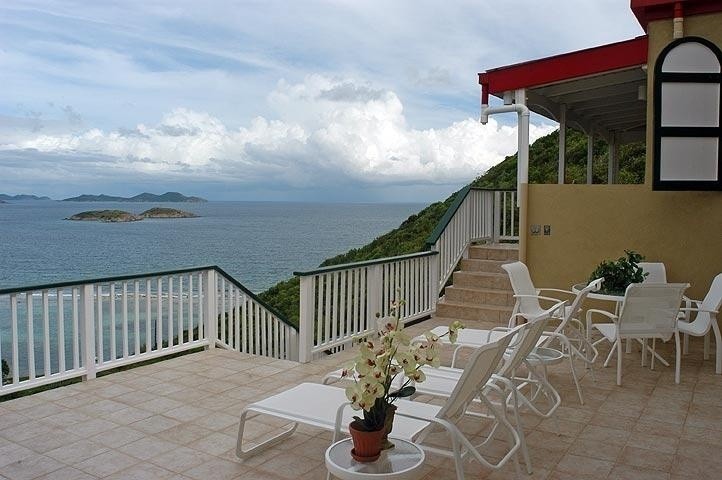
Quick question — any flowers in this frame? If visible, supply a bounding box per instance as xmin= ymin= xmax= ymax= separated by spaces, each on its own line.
xmin=340 ymin=286 xmax=463 ymax=412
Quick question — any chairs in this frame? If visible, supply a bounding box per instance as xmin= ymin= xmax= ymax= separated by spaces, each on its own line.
xmin=406 ymin=297 xmax=598 ymax=406
xmin=501 ymin=261 xmax=586 ymax=356
xmin=236 ymin=322 xmax=524 ymax=478
xmin=570 ymin=259 xmax=722 ymax=389
xmin=322 ymin=315 xmax=563 ymax=472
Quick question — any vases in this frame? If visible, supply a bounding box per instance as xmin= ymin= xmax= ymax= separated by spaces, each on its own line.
xmin=344 ymin=404 xmax=399 ymax=462
